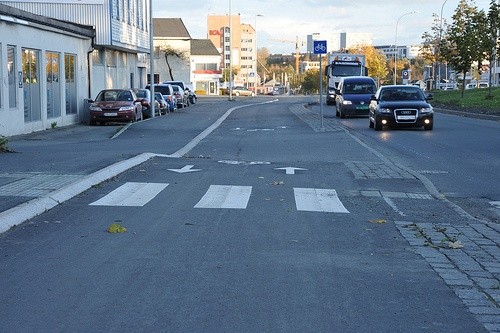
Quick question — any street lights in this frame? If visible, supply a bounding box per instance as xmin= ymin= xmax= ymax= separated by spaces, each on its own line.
xmin=394 ymin=12 xmax=415 ymax=85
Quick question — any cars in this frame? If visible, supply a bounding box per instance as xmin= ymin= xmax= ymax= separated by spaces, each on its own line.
xmin=116 ymin=80 xmax=198 ymax=120
xmin=369 ymin=84 xmax=434 ymax=131
xmin=87 ymin=88 xmax=146 ymax=126
xmin=226 ymin=86 xmax=255 ymax=98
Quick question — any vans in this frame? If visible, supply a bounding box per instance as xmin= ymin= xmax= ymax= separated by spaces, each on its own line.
xmin=334 ymin=76 xmax=378 ymax=119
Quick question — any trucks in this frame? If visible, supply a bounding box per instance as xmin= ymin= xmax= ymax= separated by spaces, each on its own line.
xmin=323 ymin=52 xmax=369 ymax=105
